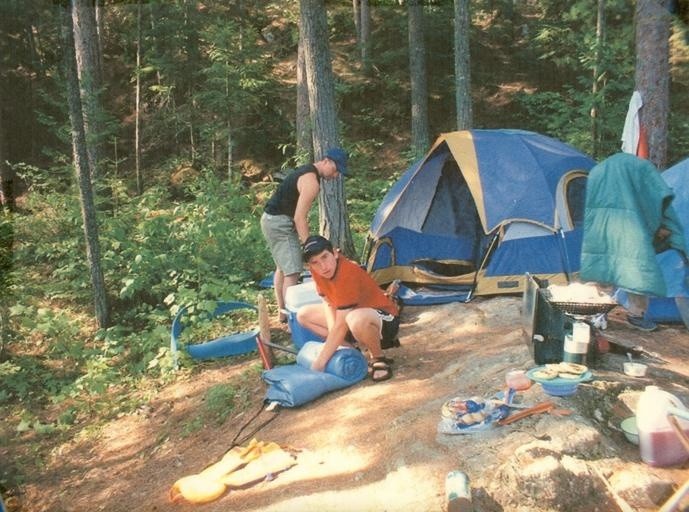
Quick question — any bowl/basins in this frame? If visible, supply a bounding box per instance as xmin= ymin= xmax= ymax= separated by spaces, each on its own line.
xmin=623 ymin=362 xmax=648 ymax=377
xmin=620 ymin=415 xmax=639 ymax=448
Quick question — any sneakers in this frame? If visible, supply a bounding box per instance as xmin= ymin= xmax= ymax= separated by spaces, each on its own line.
xmin=628 ymin=316 xmax=657 ymax=331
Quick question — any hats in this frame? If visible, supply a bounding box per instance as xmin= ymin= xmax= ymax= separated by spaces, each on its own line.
xmin=303 ymin=236 xmax=323 ymax=263
xmin=325 ymin=149 xmax=351 ymax=178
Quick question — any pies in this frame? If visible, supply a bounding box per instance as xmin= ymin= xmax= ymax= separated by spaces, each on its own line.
xmin=533 ymin=361 xmax=588 ymax=380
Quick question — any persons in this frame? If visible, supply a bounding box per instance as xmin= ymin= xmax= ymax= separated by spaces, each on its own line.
xmin=295 ymin=233 xmax=403 ymax=385
xmin=259 ymin=147 xmax=356 ymax=322
xmin=618 ymin=224 xmax=688 ymax=332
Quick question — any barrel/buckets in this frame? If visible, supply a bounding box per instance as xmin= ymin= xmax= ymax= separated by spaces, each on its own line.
xmin=635 ymin=385 xmax=689 ymax=466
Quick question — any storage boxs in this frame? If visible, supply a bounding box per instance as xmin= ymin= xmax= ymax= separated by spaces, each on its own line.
xmin=284 ymin=281 xmax=325 ymax=350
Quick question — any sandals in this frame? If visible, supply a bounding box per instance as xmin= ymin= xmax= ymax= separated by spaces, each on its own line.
xmin=368 ymin=355 xmax=394 ymax=380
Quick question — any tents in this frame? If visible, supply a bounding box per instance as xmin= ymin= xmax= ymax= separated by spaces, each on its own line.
xmin=361 ymin=122 xmax=606 ymax=306
xmin=611 ymin=157 xmax=689 ymax=327
